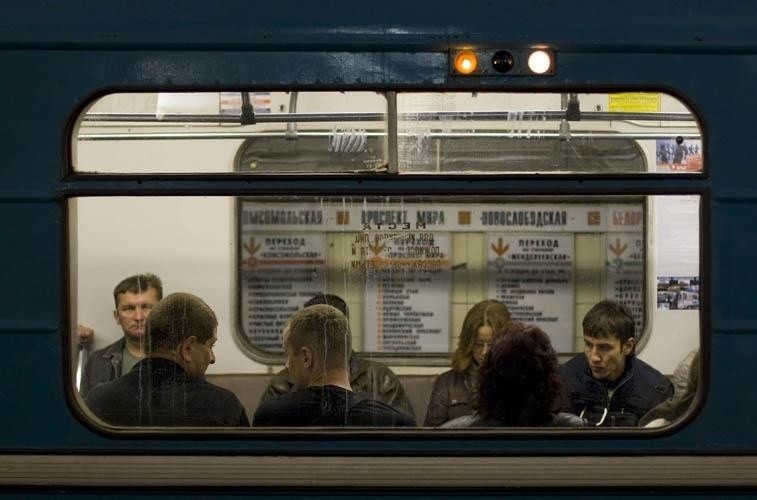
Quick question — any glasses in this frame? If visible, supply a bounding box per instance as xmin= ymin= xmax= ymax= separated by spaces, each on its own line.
xmin=471 ymin=339 xmax=496 ymax=349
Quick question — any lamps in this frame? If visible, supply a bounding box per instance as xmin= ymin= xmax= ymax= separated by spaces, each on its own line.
xmin=447 ymin=48 xmax=559 ymax=77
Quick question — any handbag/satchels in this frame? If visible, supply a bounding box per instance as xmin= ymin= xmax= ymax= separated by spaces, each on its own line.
xmin=578 ymin=406 xmax=636 ymax=427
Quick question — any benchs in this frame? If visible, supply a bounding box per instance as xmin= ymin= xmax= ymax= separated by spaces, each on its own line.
xmin=202 ymin=373 xmax=438 ymax=427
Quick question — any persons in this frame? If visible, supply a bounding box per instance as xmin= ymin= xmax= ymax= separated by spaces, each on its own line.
xmin=558 ymin=300 xmax=674 ymax=426
xmin=638 ymin=348 xmax=699 ymax=426
xmin=253 ymin=304 xmax=418 ymax=427
xmin=79 ymin=272 xmax=162 ymax=399
xmin=84 ymin=292 xmax=250 ymax=426
xmin=439 ymin=322 xmax=584 ymax=427
xmin=257 ymin=294 xmax=415 ymax=417
xmin=423 ymin=299 xmax=511 ymax=426
